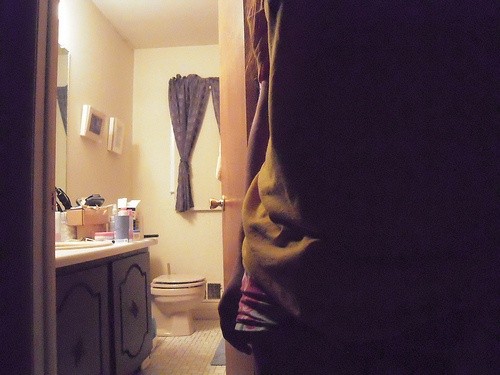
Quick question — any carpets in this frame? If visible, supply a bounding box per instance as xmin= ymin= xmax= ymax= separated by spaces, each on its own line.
xmin=210 ymin=337 xmax=226 ymax=366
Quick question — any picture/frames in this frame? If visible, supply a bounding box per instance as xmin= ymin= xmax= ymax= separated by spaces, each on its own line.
xmin=79 ymin=104 xmax=107 ymax=143
xmin=107 ymin=117 xmax=125 ymax=154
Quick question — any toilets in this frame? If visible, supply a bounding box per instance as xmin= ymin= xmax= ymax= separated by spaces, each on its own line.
xmin=150 ymin=275 xmax=206 ymax=337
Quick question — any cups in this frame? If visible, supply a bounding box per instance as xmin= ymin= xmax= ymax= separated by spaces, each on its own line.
xmin=114 ymin=215 xmax=129 ymax=242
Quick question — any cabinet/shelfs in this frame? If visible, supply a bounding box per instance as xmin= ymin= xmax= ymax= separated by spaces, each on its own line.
xmin=55 ymin=247 xmax=154 ymax=374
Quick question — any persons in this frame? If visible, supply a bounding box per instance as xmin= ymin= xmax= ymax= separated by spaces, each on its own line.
xmin=219 ymin=1 xmax=500 ymax=375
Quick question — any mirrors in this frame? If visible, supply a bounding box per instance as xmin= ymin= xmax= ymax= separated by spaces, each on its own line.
xmin=56 ymin=43 xmax=70 ymax=211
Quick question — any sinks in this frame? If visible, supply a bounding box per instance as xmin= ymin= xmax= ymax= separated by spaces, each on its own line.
xmin=55 ymin=241 xmax=113 ymax=250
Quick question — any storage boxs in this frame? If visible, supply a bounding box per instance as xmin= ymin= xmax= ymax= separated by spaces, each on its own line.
xmin=77 ymin=224 xmax=105 ymax=238
xmin=66 ymin=207 xmax=108 ymax=226
